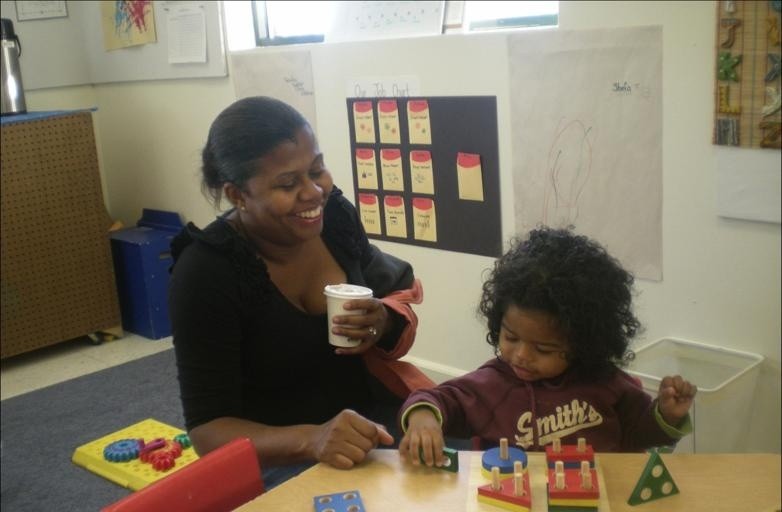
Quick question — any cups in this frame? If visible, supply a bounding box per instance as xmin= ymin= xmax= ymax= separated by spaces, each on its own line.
xmin=322 ymin=283 xmax=376 ymax=350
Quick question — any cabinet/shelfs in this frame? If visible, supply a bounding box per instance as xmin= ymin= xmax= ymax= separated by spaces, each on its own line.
xmin=0 ymin=107 xmax=122 ymax=361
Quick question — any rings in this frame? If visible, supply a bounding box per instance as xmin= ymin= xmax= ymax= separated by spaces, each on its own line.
xmin=367 ymin=326 xmax=378 ymax=338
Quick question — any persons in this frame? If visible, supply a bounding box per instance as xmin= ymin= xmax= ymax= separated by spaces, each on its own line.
xmin=399 ymin=226 xmax=697 ymax=467
xmin=170 ymin=98 xmax=438 ymax=490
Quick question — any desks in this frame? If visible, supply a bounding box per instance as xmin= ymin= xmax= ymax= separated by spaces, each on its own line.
xmin=232 ymin=448 xmax=782 ymax=512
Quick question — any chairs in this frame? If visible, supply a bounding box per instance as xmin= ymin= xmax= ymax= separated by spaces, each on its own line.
xmin=94 ymin=438 xmax=268 ymax=512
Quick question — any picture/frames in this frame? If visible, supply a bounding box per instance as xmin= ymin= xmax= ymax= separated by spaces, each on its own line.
xmin=14 ymin=0 xmax=69 ymax=22
